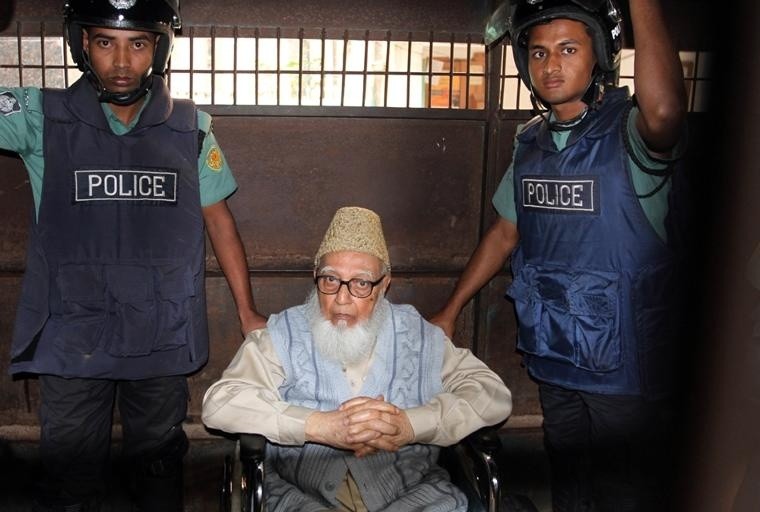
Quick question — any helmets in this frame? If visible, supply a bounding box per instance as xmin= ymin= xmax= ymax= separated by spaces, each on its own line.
xmin=510 ymin=1 xmax=624 ymax=91
xmin=62 ymin=1 xmax=182 ymax=73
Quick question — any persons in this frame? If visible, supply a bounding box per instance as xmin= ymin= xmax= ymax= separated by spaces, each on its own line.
xmin=201 ymin=206 xmax=513 ymax=512
xmin=0 ymin=0 xmax=268 ymax=512
xmin=429 ymin=1 xmax=689 ymax=512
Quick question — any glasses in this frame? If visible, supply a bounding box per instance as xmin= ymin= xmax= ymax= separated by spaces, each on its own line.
xmin=314 ymin=272 xmax=387 ymax=299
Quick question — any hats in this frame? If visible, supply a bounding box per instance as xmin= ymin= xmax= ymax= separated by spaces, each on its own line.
xmin=314 ymin=206 xmax=393 ymax=273
